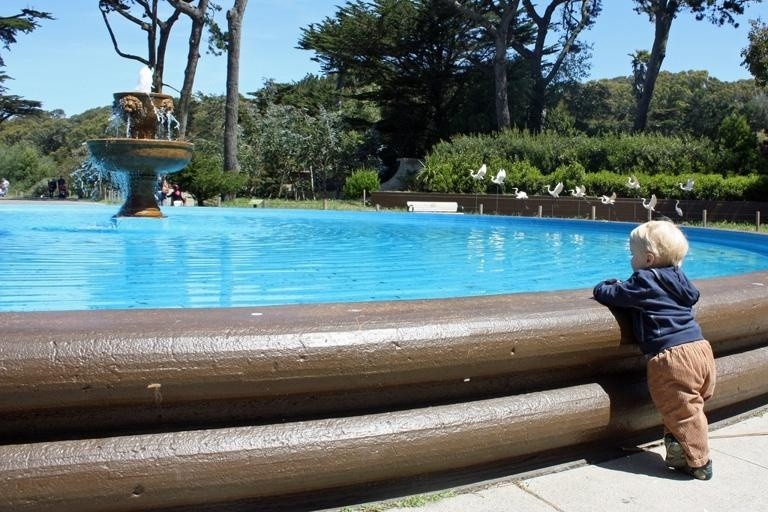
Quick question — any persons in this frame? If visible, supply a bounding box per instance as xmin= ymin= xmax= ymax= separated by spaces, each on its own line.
xmin=0 ymin=177 xmax=10 ymax=197
xmin=592 ymin=219 xmax=719 ymax=482
xmin=46 ymin=171 xmax=186 ymax=206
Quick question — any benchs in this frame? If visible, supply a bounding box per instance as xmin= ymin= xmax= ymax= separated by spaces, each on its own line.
xmin=406 ymin=201 xmax=465 ymax=214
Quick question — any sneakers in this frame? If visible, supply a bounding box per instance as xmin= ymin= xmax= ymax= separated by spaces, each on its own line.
xmin=684 ymin=460 xmax=712 ymax=480
xmin=665 ymin=434 xmax=687 ymax=469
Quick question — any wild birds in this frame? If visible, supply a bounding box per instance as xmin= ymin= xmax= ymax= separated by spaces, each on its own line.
xmin=640 ymin=194 xmax=658 ymax=212
xmin=544 ymin=182 xmax=564 ymax=204
xmin=512 ymin=187 xmax=528 ymax=208
xmin=675 ymin=199 xmax=684 ymax=220
xmin=467 ymin=163 xmax=487 ymax=184
xmin=488 ymin=169 xmax=507 ymax=190
xmin=678 ymin=178 xmax=695 ymax=192
xmin=567 ymin=185 xmax=587 ymax=200
xmin=598 ymin=191 xmax=617 ymax=210
xmin=624 ymin=175 xmax=640 ymax=194
